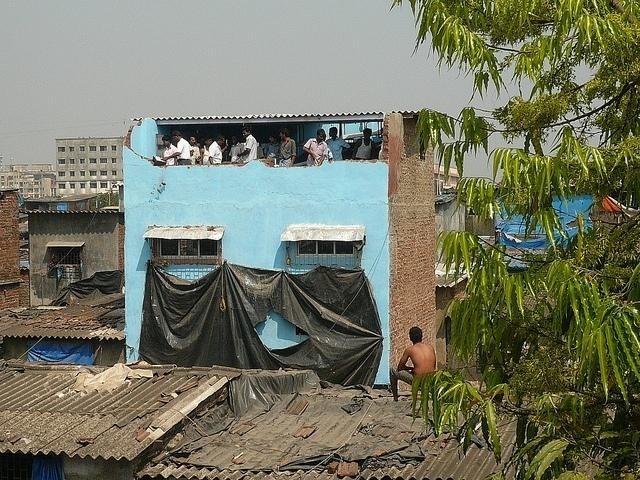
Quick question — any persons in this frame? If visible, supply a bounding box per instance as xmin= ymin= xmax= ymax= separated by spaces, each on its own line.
xmin=389 ymin=327 xmax=437 ymax=403
xmin=161 ymin=125 xmax=376 ymax=166
